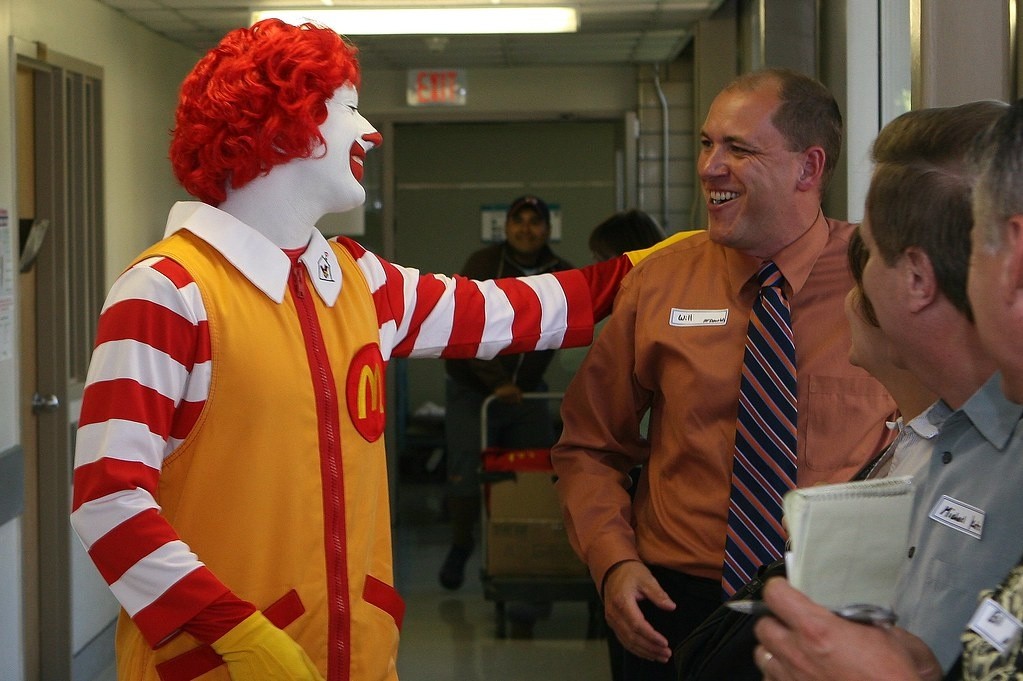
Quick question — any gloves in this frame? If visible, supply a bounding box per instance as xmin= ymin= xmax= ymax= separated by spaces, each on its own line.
xmin=209 ymin=609 xmax=326 ymax=681
xmin=622 ymin=230 xmax=707 ymax=267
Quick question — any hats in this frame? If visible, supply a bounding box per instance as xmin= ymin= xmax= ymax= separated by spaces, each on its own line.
xmin=508 ymin=196 xmax=547 ymax=216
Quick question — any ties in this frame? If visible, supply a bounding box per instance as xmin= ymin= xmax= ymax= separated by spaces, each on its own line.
xmin=720 ymin=260 xmax=799 ymax=600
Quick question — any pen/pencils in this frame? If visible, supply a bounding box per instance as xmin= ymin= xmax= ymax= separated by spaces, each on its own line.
xmin=725 ymin=600 xmax=775 ymax=616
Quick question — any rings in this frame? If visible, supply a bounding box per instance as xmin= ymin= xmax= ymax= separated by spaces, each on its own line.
xmin=759 ymin=653 xmax=772 ymax=675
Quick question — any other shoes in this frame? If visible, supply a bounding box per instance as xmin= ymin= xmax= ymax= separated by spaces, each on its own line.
xmin=439 ymin=534 xmax=475 ymax=591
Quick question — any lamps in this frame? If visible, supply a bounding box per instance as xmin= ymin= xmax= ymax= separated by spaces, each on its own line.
xmin=244 ymin=3 xmax=581 ymax=34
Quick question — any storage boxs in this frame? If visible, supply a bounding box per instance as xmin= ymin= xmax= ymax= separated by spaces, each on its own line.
xmin=485 ymin=470 xmax=590 ymax=576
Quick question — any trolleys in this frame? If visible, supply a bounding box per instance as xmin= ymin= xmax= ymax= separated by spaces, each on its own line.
xmin=476 ymin=388 xmax=616 ymax=643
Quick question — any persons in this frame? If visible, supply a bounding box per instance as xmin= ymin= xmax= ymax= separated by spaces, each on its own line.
xmin=550 ymin=66 xmax=1023 ymax=681
xmin=438 ymin=599 xmax=605 ymax=681
xmin=436 ymin=197 xmax=573 ymax=589
xmin=69 ymin=18 xmax=704 ymax=681
